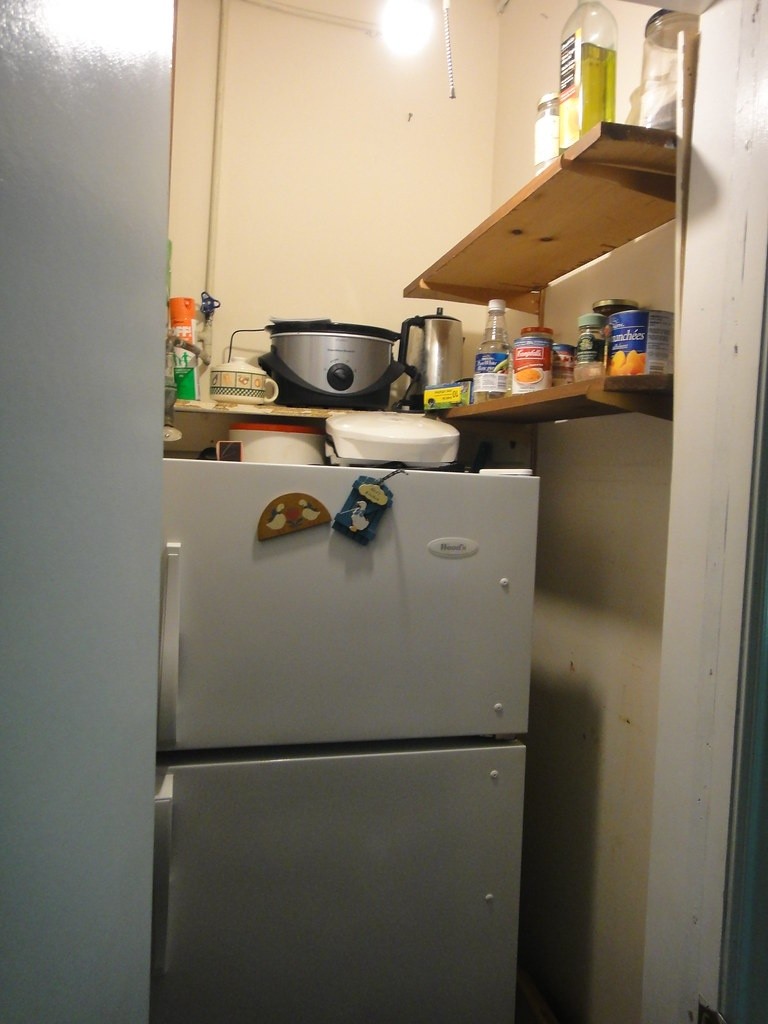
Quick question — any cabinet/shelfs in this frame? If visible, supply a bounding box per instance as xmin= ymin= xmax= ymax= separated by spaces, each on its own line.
xmin=402 ymin=122 xmax=676 ymax=423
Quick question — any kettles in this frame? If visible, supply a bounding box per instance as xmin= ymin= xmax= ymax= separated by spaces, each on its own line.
xmin=398 ymin=306 xmax=465 ymax=409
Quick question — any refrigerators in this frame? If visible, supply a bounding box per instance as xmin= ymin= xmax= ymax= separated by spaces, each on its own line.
xmin=150 ymin=457 xmax=539 ymax=1024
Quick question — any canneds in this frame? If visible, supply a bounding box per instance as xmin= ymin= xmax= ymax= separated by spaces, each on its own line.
xmin=509 ymin=299 xmax=677 ymax=395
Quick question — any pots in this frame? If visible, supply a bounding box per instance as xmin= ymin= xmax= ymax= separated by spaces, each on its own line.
xmin=258 ymin=320 xmax=404 ymax=408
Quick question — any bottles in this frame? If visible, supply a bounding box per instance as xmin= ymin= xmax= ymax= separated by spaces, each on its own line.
xmin=534 ymin=93 xmax=560 ymax=177
xmin=561 ymin=0 xmax=618 ymax=156
xmin=573 ymin=314 xmax=606 ymax=379
xmin=474 ymin=300 xmax=511 ymax=404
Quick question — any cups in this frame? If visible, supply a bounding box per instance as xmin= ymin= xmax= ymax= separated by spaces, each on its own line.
xmin=641 ymin=9 xmax=696 ymax=129
xmin=209 ymin=356 xmax=278 ymax=403
